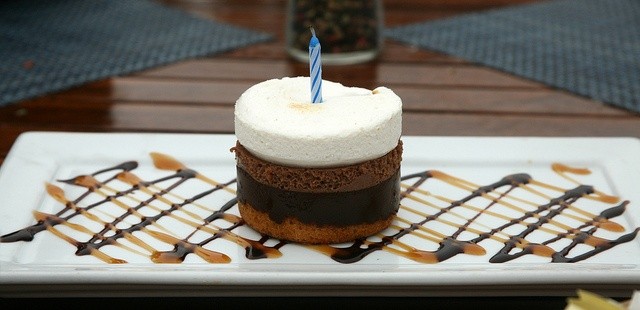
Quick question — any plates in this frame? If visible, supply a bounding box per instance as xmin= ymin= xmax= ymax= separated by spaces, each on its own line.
xmin=1 ymin=130 xmax=640 ymax=298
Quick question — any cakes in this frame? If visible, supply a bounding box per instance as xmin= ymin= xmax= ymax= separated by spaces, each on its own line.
xmin=230 ymin=75 xmax=404 ymax=246
